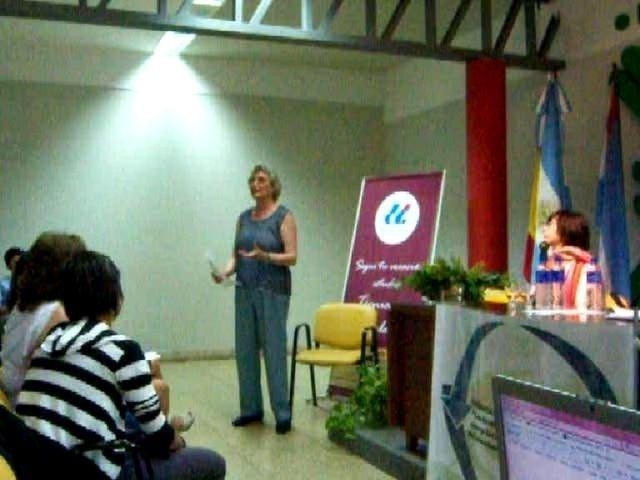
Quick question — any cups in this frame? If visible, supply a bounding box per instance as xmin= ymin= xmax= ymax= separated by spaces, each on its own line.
xmin=141 ymin=351 xmax=162 ymax=379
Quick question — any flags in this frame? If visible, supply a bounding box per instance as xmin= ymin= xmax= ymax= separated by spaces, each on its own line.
xmin=519 ymin=68 xmax=573 ymax=305
xmin=592 ymin=67 xmax=633 ymax=309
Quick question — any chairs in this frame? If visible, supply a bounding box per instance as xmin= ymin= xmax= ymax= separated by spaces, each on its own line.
xmin=0 ymin=388 xmax=155 ymax=480
xmin=289 ymin=302 xmax=378 ymax=421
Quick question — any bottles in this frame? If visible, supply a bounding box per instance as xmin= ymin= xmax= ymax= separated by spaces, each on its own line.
xmin=533 ymin=253 xmax=551 ymax=308
xmin=585 ymin=254 xmax=605 ymax=311
xmin=551 ymin=254 xmax=566 ymax=310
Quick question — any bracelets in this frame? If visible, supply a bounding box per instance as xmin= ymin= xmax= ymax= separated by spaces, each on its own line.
xmin=263 ymin=251 xmax=272 ymax=265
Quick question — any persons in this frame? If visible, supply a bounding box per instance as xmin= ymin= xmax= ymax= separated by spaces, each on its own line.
xmin=528 ymin=207 xmax=606 ymax=314
xmin=209 ymin=161 xmax=298 ymax=435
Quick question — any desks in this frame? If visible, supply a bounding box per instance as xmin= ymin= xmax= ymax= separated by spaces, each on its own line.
xmin=386 ymin=302 xmax=639 ymax=451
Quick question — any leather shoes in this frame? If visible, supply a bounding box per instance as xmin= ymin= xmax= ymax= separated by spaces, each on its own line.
xmin=231 ymin=410 xmax=263 ymax=427
xmin=275 ymin=419 xmax=291 ymax=434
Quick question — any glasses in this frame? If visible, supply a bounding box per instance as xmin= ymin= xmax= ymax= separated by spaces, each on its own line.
xmin=249 ymin=177 xmax=266 ymax=182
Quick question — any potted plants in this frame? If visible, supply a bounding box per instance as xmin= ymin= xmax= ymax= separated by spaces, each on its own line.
xmin=406 ymin=256 xmax=511 ymax=307
xmin=326 ymin=363 xmax=388 ymax=436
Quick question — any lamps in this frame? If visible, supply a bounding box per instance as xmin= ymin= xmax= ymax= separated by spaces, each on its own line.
xmin=153 ymin=0 xmax=226 ymax=53
